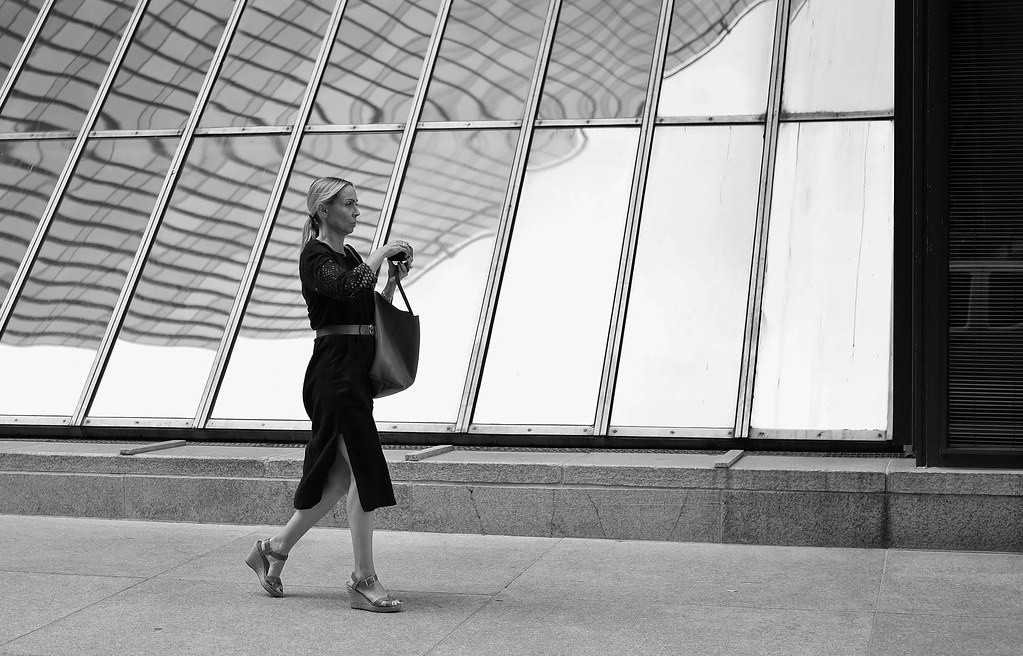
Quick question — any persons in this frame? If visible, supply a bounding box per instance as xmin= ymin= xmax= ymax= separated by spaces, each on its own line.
xmin=245 ymin=176 xmax=414 ymax=612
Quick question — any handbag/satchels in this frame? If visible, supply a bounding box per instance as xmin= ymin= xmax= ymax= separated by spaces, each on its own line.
xmin=367 ymin=267 xmax=420 ymax=399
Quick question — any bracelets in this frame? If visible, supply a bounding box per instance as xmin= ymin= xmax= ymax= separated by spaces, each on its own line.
xmin=382 ymin=290 xmax=394 ymax=300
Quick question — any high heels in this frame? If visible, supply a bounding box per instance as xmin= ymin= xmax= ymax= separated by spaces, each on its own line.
xmin=346 ymin=572 xmax=403 ymax=612
xmin=245 ymin=538 xmax=288 ymax=599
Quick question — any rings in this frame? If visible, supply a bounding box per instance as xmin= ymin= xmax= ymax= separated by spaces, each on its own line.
xmin=407 ymin=266 xmax=413 ymax=269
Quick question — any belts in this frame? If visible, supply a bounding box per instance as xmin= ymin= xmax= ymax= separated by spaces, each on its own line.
xmin=316 ymin=323 xmax=374 ymax=336
